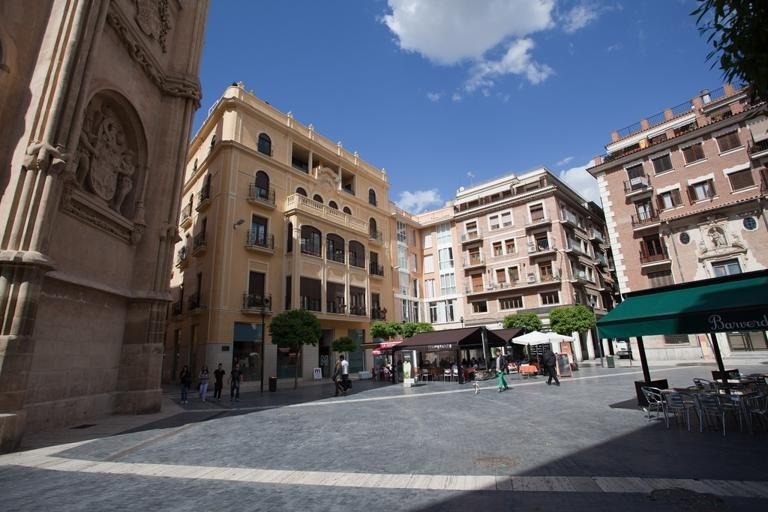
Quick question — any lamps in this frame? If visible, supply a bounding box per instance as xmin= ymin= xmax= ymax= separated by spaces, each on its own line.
xmin=234 ymin=219 xmax=244 ymax=229
xmin=392 ymin=265 xmax=401 ymax=270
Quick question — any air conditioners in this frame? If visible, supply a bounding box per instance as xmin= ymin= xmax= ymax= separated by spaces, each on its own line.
xmin=485 ymin=246 xmax=536 ymax=290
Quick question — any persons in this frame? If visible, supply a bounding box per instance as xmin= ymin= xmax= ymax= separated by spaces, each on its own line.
xmin=196 ymin=363 xmax=210 ymax=403
xmin=229 ymin=361 xmax=244 ymax=402
xmin=708 ymin=227 xmax=721 ymax=248
xmin=542 ymin=344 xmax=561 ymax=387
xmin=72 ymin=115 xmax=101 ymax=191
xmin=371 ymin=353 xmax=547 ymax=384
xmin=330 ymin=359 xmax=347 ymax=397
xmin=179 ymin=365 xmax=191 ymax=404
xmin=108 ymin=147 xmax=136 ymax=216
xmin=493 ymin=348 xmax=509 ymax=393
xmin=338 ymin=354 xmax=350 ymax=396
xmin=213 ymin=362 xmax=225 ymax=401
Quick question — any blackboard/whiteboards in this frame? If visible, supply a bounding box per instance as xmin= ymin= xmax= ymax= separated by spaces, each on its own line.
xmin=556 ymin=354 xmax=571 ymax=376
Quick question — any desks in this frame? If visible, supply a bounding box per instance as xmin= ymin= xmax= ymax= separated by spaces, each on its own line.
xmin=519 ymin=365 xmax=539 ymax=380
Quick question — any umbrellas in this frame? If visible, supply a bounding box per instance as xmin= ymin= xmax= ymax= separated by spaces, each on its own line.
xmin=509 ymin=330 xmax=551 ymax=369
xmin=543 ymin=332 xmax=575 ymax=343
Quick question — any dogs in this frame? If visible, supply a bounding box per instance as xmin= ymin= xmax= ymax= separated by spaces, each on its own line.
xmin=471 ymin=380 xmax=480 ymax=395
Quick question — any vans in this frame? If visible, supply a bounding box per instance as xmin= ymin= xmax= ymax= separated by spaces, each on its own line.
xmin=612 ymin=336 xmax=632 ymax=358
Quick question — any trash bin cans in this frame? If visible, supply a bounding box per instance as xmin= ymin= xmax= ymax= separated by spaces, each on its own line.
xmin=606 ymin=356 xmax=615 ymax=368
xmin=269 ymin=377 xmax=278 ymax=392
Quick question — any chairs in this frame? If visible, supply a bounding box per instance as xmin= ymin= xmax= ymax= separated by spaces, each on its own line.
xmin=641 ymin=374 xmax=768 ymax=437
xmin=372 ymin=360 xmax=531 ymax=384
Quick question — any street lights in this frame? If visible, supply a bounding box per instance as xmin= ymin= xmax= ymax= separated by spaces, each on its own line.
xmin=459 ymin=316 xmax=465 ymax=359
xmin=261 ymin=294 xmax=269 ymax=394
